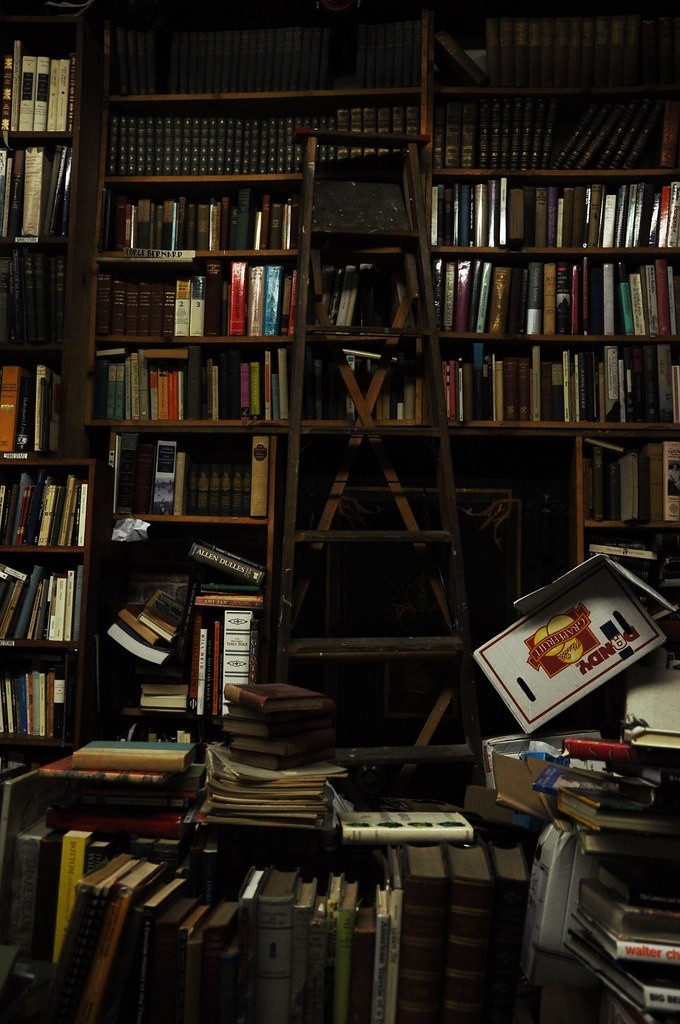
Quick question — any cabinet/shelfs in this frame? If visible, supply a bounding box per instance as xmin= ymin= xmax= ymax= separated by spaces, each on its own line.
xmin=0 ymin=0 xmax=680 ymax=761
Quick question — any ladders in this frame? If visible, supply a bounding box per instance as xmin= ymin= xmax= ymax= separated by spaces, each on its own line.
xmin=274 ymin=128 xmax=486 ymax=787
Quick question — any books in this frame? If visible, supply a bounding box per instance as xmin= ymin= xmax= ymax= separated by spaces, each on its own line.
xmin=224 ymin=680 xmax=335 ymax=713
xmin=0 ymin=0 xmax=680 ymax=1024
